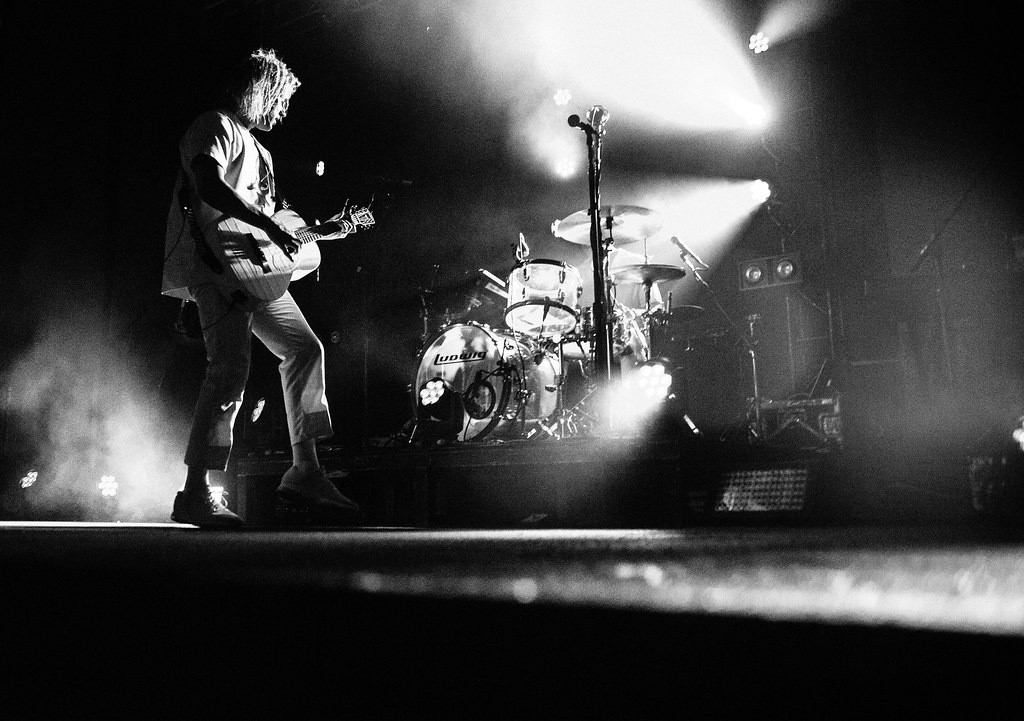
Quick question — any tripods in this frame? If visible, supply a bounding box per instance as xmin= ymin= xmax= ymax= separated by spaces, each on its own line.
xmin=678 ymin=254 xmax=829 ymax=449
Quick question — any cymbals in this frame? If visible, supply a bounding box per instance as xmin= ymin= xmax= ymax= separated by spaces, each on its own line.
xmin=668 ymin=305 xmax=707 ymax=321
xmin=557 ymin=204 xmax=663 ymax=244
xmin=608 ymin=263 xmax=687 ymax=285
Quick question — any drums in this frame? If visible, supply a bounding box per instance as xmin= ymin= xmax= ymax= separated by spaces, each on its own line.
xmin=563 ymin=308 xmax=630 ymax=362
xmin=409 ymin=321 xmax=561 ymax=444
xmin=504 ymin=258 xmax=584 ymax=341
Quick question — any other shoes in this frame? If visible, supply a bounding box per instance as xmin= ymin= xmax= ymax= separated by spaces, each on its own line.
xmin=171 ymin=490 xmax=248 ymax=532
xmin=273 ymin=466 xmax=359 ymax=513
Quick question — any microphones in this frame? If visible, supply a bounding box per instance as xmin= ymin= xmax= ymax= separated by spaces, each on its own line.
xmin=586 ymin=105 xmax=610 ymax=125
xmin=671 ymin=236 xmax=708 ymax=268
xmin=568 ymin=114 xmax=601 ymax=138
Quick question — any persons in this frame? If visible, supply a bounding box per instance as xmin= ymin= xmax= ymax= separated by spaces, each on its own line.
xmin=162 ymin=49 xmax=363 ymax=534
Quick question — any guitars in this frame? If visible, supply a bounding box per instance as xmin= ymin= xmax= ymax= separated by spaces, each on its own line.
xmin=204 ymin=198 xmax=377 ymax=314
